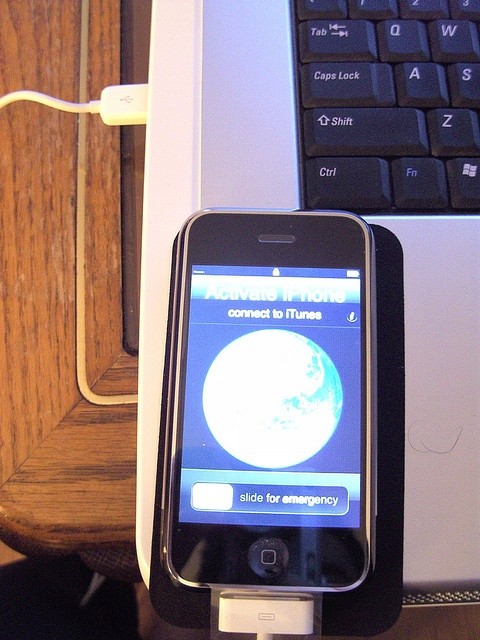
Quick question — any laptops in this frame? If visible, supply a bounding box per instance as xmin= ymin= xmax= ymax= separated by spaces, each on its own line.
xmin=132 ymin=0 xmax=478 ymax=604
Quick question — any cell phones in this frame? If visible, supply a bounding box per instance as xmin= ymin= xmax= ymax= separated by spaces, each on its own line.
xmin=161 ymin=208 xmax=381 ymax=600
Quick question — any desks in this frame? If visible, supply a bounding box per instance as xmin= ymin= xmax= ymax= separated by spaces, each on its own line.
xmin=0 ymin=0 xmax=152 ymax=580
